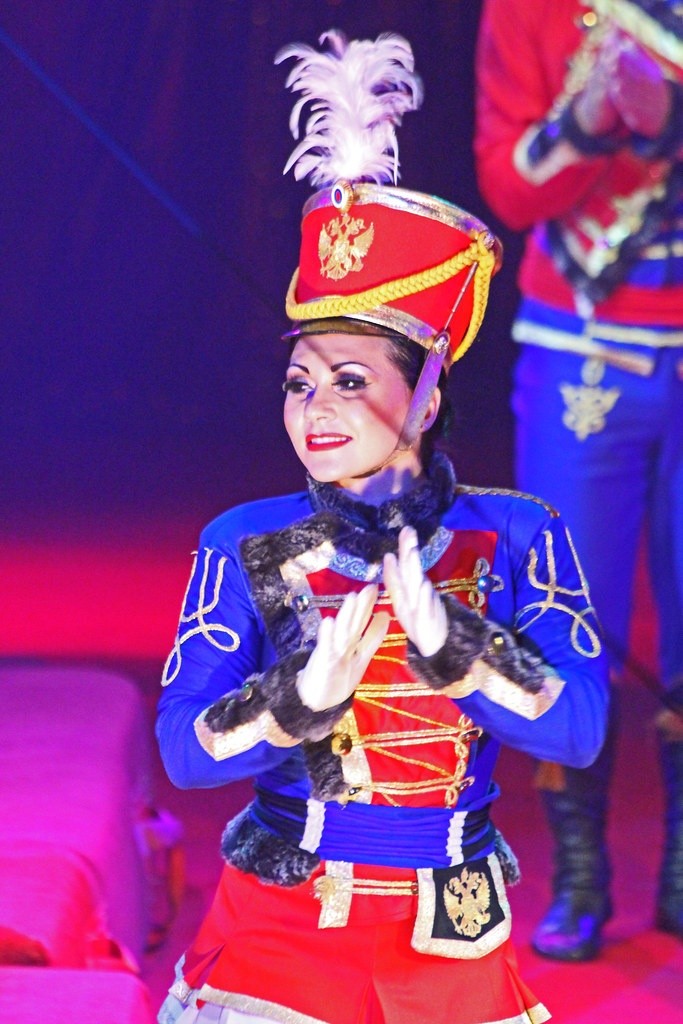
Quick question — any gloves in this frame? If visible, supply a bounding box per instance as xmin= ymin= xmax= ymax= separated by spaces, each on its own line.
xmin=383 ymin=525 xmax=450 ymax=656
xmin=296 ymin=583 xmax=391 ymax=710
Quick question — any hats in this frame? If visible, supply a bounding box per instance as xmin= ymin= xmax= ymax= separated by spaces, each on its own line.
xmin=278 ymin=25 xmax=503 ymax=391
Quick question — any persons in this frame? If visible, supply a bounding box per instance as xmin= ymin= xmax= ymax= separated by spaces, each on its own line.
xmin=472 ymin=1 xmax=683 ymax=963
xmin=151 ymin=304 xmax=616 ymax=1024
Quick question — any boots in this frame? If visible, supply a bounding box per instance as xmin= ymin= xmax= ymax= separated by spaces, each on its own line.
xmin=659 ymin=727 xmax=683 ymax=931
xmin=531 ymin=681 xmax=620 ymax=962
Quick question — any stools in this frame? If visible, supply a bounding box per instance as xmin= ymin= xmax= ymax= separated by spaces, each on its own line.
xmin=0 ymin=665 xmax=188 ymax=972
xmin=0 ymin=966 xmax=158 ymax=1024
